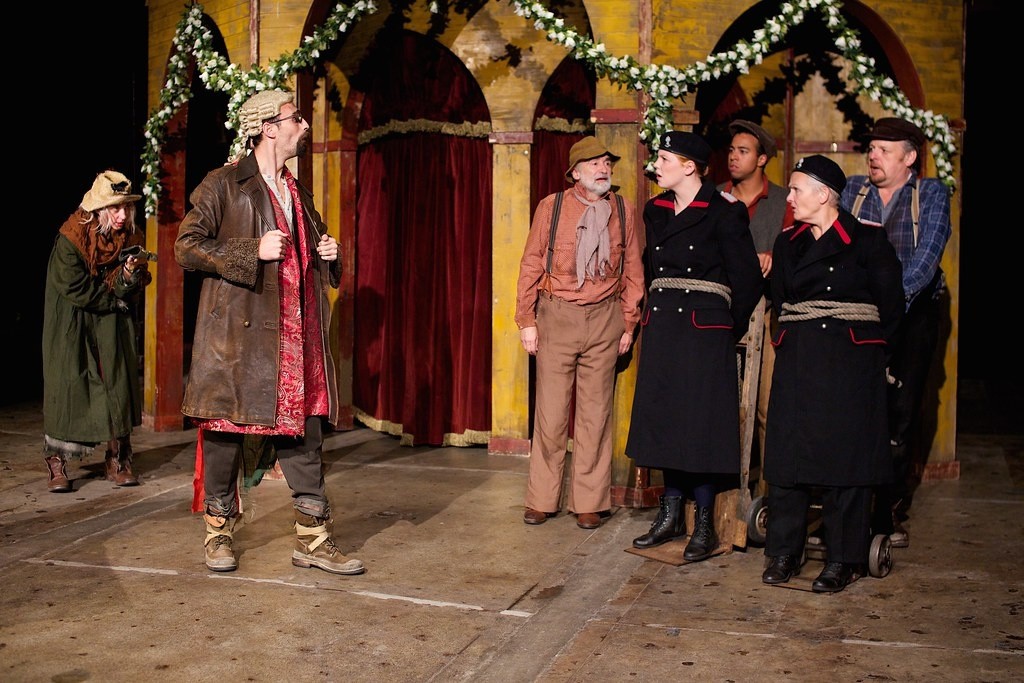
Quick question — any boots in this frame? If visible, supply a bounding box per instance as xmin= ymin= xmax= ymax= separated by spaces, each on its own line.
xmin=633 ymin=495 xmax=687 ymax=548
xmin=293 ymin=508 xmax=365 ymax=576
xmin=202 ymin=514 xmax=237 ymax=571
xmin=875 ymin=499 xmax=908 ymax=547
xmin=683 ymin=505 xmax=717 ymax=561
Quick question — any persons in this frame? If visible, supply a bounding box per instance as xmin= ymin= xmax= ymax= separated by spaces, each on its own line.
xmin=514 ymin=136 xmax=646 ymax=527
xmin=44 ymin=170 xmax=152 ymax=491
xmin=174 ymin=91 xmax=364 ymax=574
xmin=625 ymin=131 xmax=761 ymax=559
xmin=760 ymin=155 xmax=906 ymax=594
xmin=841 ymin=117 xmax=952 ymax=548
xmin=713 ymin=120 xmax=795 ymax=497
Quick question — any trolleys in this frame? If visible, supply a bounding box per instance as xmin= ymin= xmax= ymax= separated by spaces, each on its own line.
xmin=621 ymin=296 xmax=774 ymax=566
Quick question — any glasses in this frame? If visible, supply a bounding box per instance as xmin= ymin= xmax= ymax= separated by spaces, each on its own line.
xmin=268 ymin=110 xmax=304 ymax=124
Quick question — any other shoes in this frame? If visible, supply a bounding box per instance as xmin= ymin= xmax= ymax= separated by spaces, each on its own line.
xmin=524 ymin=510 xmax=547 ymax=524
xmin=577 ymin=513 xmax=601 ymax=529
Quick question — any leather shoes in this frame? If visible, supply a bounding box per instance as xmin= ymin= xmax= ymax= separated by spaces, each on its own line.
xmin=104 ymin=453 xmax=138 ymax=486
xmin=763 ymin=553 xmax=800 ymax=585
xmin=44 ymin=456 xmax=70 ymax=491
xmin=811 ymin=561 xmax=860 ymax=591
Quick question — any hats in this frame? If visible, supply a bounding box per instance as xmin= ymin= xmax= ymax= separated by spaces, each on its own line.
xmin=80 ymin=170 xmax=141 ymax=214
xmin=730 ymin=120 xmax=777 ymax=160
xmin=659 ymin=130 xmax=710 ymax=163
xmin=566 ymin=135 xmax=622 ymax=185
xmin=866 ymin=117 xmax=925 ymax=155
xmin=793 ymin=154 xmax=848 ymax=197
